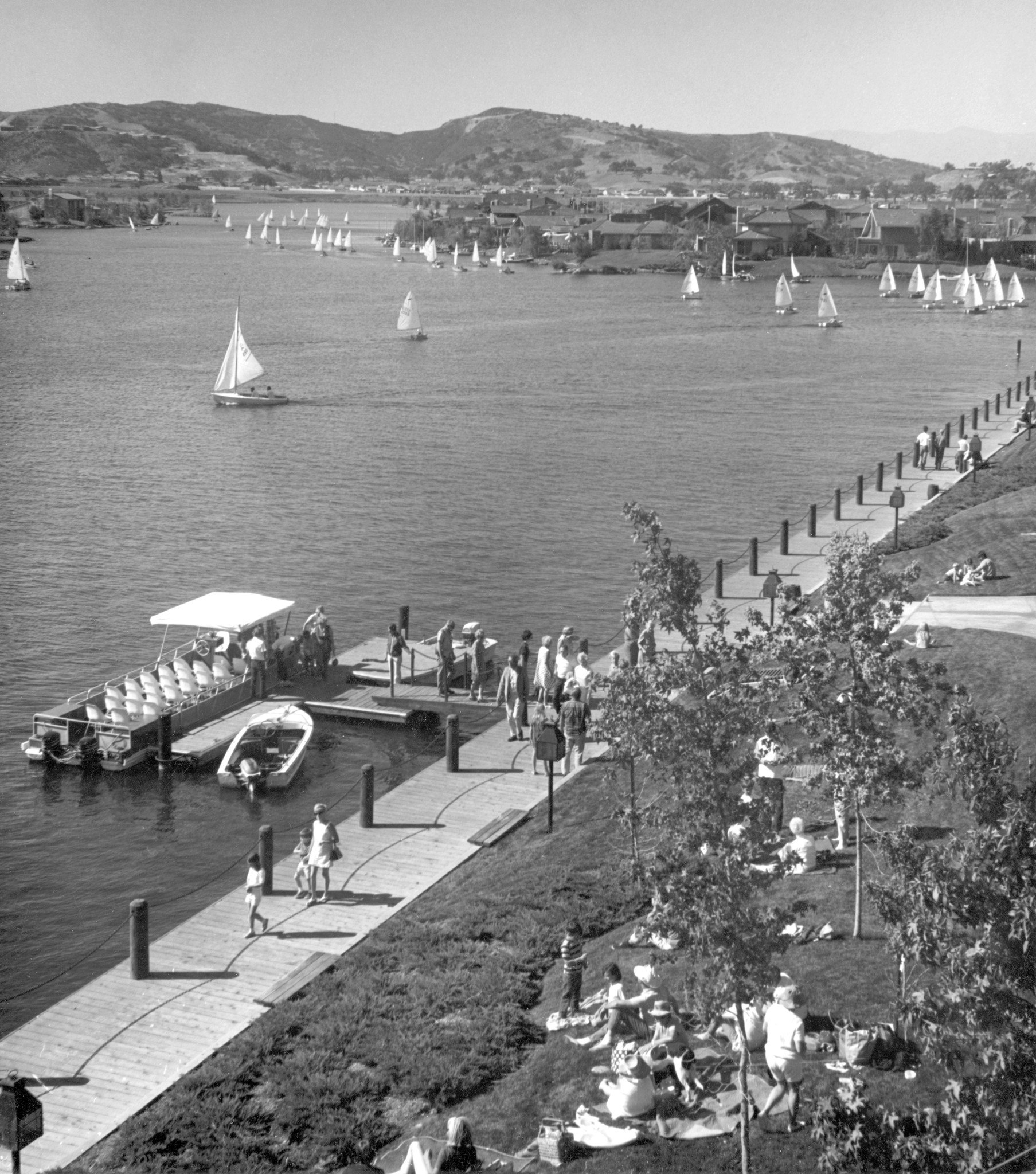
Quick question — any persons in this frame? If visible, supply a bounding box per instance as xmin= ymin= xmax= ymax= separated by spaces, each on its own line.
xmin=385 ymin=620 xmax=656 ymax=776
xmin=915 ymin=426 xmax=973 ymax=473
xmin=416 ymin=329 xmax=423 ymax=336
xmin=249 ymin=386 xmax=276 ymax=398
xmin=559 ymin=886 xmax=807 ymax=1134
xmin=245 ymin=605 xmax=336 ymax=699
xmin=293 ymin=803 xmax=339 ymax=907
xmin=934 ymin=551 xmax=996 ymax=587
xmin=1011 ymin=407 xmax=1031 ymax=432
xmin=393 ymin=1116 xmax=482 ymax=1174
xmin=456 ymin=261 xmax=511 ymax=272
xmin=883 ymin=290 xmax=981 ymax=311
xmin=902 ymin=623 xmax=937 ymax=649
xmin=722 ymin=720 xmax=849 ymax=874
xmin=242 ymin=852 xmax=268 ymax=939
xmin=781 ymin=305 xmax=838 ymax=324
xmin=837 ymin=672 xmax=871 ymax=704
xmin=341 ymin=1138 xmax=385 ymax=1174
xmin=933 ymin=429 xmax=945 ymax=470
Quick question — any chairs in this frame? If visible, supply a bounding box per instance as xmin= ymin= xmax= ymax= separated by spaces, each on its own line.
xmin=241 ymin=737 xmax=266 ymax=759
xmin=277 ymin=737 xmax=298 ymax=762
xmin=84 ymin=655 xmax=249 ymax=739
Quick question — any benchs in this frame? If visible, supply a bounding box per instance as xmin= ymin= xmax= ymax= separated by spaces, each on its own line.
xmin=253 ymin=951 xmax=342 ymax=1009
xmin=467 ymin=808 xmax=529 ymax=848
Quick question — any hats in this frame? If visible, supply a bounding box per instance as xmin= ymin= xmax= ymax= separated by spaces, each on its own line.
xmin=648 ymin=1000 xmax=671 ymax=1017
xmin=618 ymin=1054 xmax=653 ymax=1079
xmin=774 ymin=985 xmax=807 ymax=1009
xmin=634 ymin=964 xmax=662 ymax=988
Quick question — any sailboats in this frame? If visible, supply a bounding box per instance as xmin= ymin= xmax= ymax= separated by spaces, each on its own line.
xmin=906 ymin=263 xmax=946 ymax=309
xmin=225 ymin=215 xmax=235 ymax=231
xmin=290 ymin=207 xmax=321 ymax=228
xmin=394 ymin=289 xmax=428 ymax=341
xmin=344 ymin=211 xmax=351 ymax=224
xmin=392 ymin=236 xmax=405 ymax=262
xmin=953 ymin=257 xmax=1026 ymax=314
xmin=311 ymin=226 xmax=356 ymax=256
xmin=419 ymin=236 xmax=443 ymax=268
xmin=5 ymin=238 xmax=33 ymax=291
xmin=211 ymin=295 xmax=289 ymax=404
xmin=680 ymin=265 xmax=703 ymax=300
xmin=245 ymin=224 xmax=284 ymax=248
xmin=127 ymin=213 xmax=160 ymax=231
xmin=452 ymin=242 xmax=468 ymax=272
xmin=719 ymin=249 xmax=755 ymax=282
xmin=472 ymin=240 xmax=487 ymax=267
xmin=496 ymin=244 xmax=515 ymax=274
xmin=211 ymin=193 xmax=222 ymax=223
xmin=816 ymin=281 xmax=843 ymax=327
xmin=789 ymin=254 xmax=809 ymax=283
xmin=257 ymin=209 xmax=289 ymax=230
xmin=878 ymin=264 xmax=897 ymax=298
xmin=316 ymin=215 xmax=328 ymax=228
xmin=774 ymin=273 xmax=798 ymax=316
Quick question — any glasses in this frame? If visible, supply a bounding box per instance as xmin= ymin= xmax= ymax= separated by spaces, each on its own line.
xmin=313 ymin=811 xmax=324 ymax=816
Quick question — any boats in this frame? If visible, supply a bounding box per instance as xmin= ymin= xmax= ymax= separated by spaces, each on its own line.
xmin=490 ymin=256 xmax=533 ymax=263
xmin=217 ymin=703 xmax=314 ymax=791
xmin=18 ymin=589 xmax=325 ymax=772
xmin=351 ymin=628 xmax=498 ymax=687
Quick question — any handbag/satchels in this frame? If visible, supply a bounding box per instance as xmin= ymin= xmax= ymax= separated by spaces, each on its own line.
xmin=331 ymin=839 xmax=343 ymax=861
xmin=828 ymin=1011 xmax=922 ymax=1072
xmin=538 ymin=1118 xmax=575 ymax=1164
xmin=611 ymin=1041 xmax=637 ymax=1075
xmin=776 ymin=921 xmax=836 ymax=944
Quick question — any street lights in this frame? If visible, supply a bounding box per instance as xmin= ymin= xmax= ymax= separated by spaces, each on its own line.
xmin=762 ymin=570 xmax=783 ymax=626
xmin=535 ymin=724 xmax=567 ymax=834
xmin=967 ymin=434 xmax=982 ymax=482
xmin=1025 ymin=396 xmax=1035 ymax=441
xmin=889 ymin=484 xmax=905 ymax=553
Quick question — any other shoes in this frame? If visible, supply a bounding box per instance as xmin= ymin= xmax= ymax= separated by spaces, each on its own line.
xmin=295 ymin=891 xmax=305 ymax=898
xmin=559 ymin=1019 xmax=568 ymax=1026
xmin=589 ymin=1045 xmax=612 ymax=1052
xmin=244 ymin=933 xmax=255 ymax=938
xmin=757 ymin=1112 xmax=773 ymax=1132
xmin=611 ymin=945 xmax=624 ymax=950
xmin=518 ymin=731 xmax=523 ymax=740
xmin=788 ymin=1121 xmax=805 ymax=1133
xmin=307 ymin=898 xmax=317 ymax=906
xmin=318 ymin=896 xmax=329 ymax=903
xmin=262 ymin=919 xmax=269 ymax=932
xmin=563 ymin=1035 xmax=580 ymax=1049
xmin=305 ymin=893 xmax=312 ymax=899
xmin=507 ymin=735 xmax=516 ymax=742
